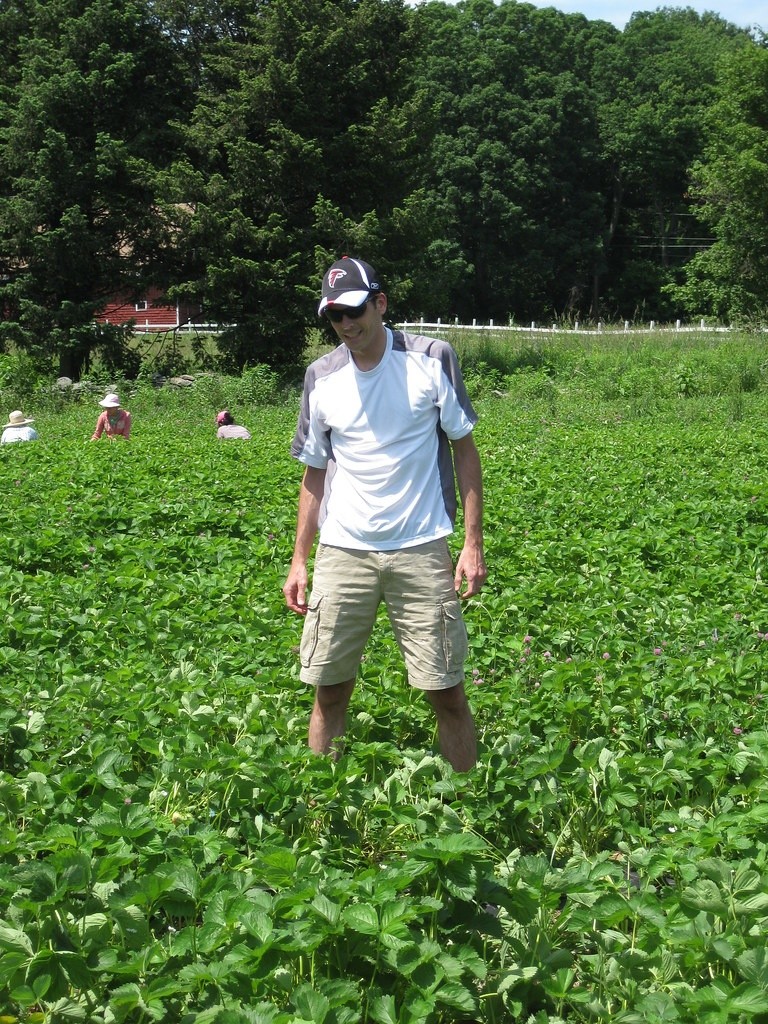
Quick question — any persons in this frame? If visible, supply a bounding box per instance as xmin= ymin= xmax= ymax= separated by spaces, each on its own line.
xmin=282 ymin=255 xmax=486 ymax=772
xmin=91 ymin=394 xmax=132 ymax=442
xmin=217 ymin=411 xmax=251 ymax=439
xmin=1 ymin=411 xmax=38 ymax=443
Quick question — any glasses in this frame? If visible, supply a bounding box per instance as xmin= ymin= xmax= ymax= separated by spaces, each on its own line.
xmin=323 ymin=294 xmax=377 ymax=323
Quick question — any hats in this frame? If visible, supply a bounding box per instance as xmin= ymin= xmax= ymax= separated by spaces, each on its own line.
xmin=217 ymin=410 xmax=232 ymax=424
xmin=2 ymin=409 xmax=36 ymax=427
xmin=317 ymin=255 xmax=381 ymax=317
xmin=99 ymin=394 xmax=121 ymax=408
